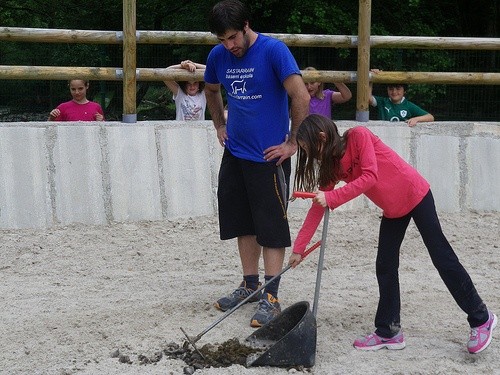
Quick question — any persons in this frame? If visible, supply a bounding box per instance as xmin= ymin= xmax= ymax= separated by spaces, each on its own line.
xmin=302 ymin=67 xmax=352 ymax=121
xmin=163 ymin=60 xmax=207 ymax=120
xmin=47 ymin=80 xmax=105 ymax=121
xmin=368 ymin=69 xmax=434 ymax=127
xmin=204 ymin=3 xmax=312 ymax=327
xmin=288 ymin=114 xmax=498 ymax=354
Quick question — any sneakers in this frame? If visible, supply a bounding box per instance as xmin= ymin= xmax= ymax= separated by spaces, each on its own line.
xmin=215 ymin=281 xmax=263 ymax=311
xmin=250 ymin=292 xmax=282 ymax=327
xmin=466 ymin=310 xmax=498 ymax=354
xmin=353 ymin=328 xmax=405 ymax=351
